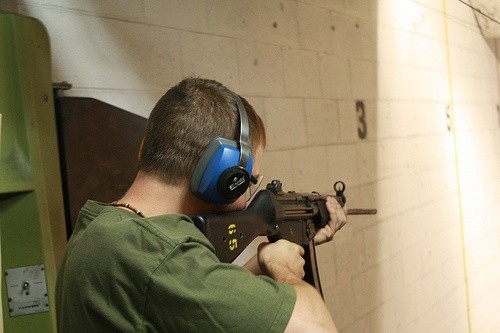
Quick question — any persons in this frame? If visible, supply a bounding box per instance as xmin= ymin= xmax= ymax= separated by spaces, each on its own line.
xmin=55 ymin=77 xmax=348 ymax=333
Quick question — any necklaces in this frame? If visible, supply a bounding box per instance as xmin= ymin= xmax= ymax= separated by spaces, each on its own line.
xmin=111 ymin=199 xmax=146 ymax=218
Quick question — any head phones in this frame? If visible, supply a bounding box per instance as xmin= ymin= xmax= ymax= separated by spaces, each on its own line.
xmin=191 ymin=93 xmax=258 ymax=204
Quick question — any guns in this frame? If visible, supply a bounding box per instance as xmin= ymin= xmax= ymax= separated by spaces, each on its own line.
xmin=192 ymin=179 xmax=378 ymax=312
